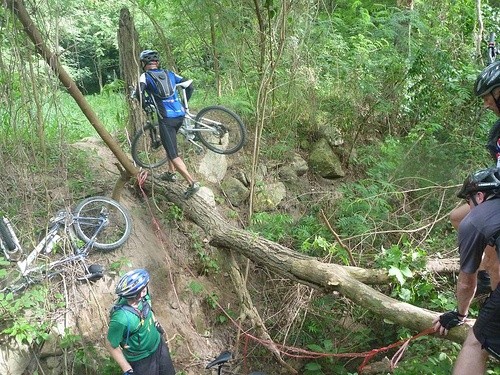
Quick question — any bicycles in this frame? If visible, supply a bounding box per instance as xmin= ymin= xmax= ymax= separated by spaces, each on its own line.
xmin=128 ymin=82 xmax=246 ymax=170
xmin=1 ymin=194 xmax=131 ymax=293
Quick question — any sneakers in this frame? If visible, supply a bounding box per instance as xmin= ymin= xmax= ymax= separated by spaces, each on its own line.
xmin=184 ymin=182 xmax=200 ymax=199
xmin=155 ymin=171 xmax=177 ymax=182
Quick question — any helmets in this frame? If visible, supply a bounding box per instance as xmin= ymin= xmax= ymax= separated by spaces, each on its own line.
xmin=457 ymin=167 xmax=500 ymax=199
xmin=115 ymin=268 xmax=151 ymax=299
xmin=139 ymin=50 xmax=159 ymax=61
xmin=473 ymin=61 xmax=500 ymax=97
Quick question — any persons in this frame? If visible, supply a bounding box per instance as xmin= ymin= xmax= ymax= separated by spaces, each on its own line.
xmin=447 ymin=59 xmax=500 ymax=293
xmin=431 ymin=167 xmax=500 ymax=374
xmin=134 ymin=48 xmax=200 ymax=200
xmin=104 ymin=267 xmax=176 ymax=375
